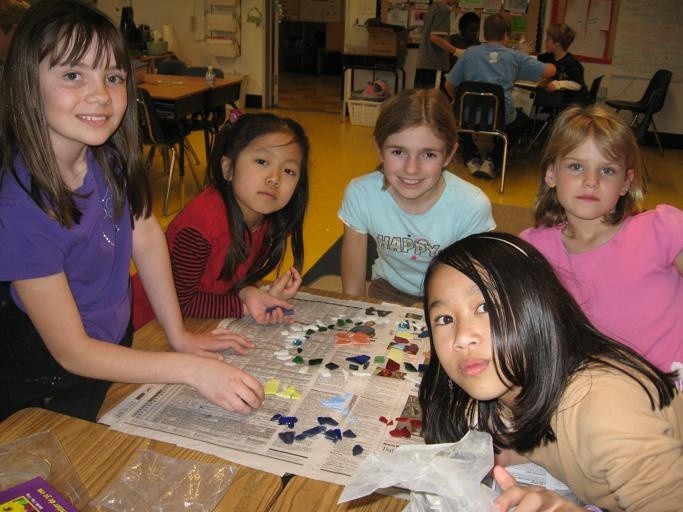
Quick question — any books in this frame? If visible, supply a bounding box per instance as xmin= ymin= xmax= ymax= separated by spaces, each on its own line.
xmin=206 ymin=1 xmax=237 ymax=46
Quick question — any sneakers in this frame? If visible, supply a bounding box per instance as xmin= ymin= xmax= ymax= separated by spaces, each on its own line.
xmin=468 ymin=159 xmax=480 ymax=178
xmin=478 ymin=159 xmax=497 ymax=177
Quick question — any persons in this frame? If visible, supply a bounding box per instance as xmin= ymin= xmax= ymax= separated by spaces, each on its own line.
xmin=336 ymin=86 xmax=497 ymax=305
xmin=130 ymin=112 xmax=310 ymax=332
xmin=418 ymin=231 xmax=683 ymax=511
xmin=412 ymin=0 xmax=465 ymax=90
xmin=0 ymin=1 xmax=267 ymax=423
xmin=485 ymin=22 xmax=590 ymax=162
xmin=518 ymin=103 xmax=683 ymax=392
xmin=443 ymin=12 xmax=557 ymax=180
xmin=440 ymin=11 xmax=482 ymax=104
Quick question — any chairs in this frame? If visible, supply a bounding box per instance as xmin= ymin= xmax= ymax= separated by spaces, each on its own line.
xmin=422 ymin=62 xmax=676 ymax=201
xmin=132 ymin=59 xmax=245 ymax=220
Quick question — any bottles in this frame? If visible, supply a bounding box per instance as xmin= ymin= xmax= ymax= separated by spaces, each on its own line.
xmin=204 ymin=66 xmax=216 ymax=85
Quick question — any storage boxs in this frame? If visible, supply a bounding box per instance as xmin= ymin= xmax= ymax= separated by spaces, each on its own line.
xmin=342 ymin=23 xmax=419 ymax=58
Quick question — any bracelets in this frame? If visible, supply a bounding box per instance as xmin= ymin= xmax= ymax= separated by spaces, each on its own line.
xmin=584 ymin=503 xmax=603 ymax=512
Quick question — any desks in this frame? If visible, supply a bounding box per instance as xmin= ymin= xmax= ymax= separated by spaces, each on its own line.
xmin=0 ymin=400 xmax=285 ymax=511
xmin=331 ymin=51 xmax=409 ymax=129
xmin=90 ymin=277 xmax=440 ymax=475
xmin=268 ymin=471 xmax=412 ymax=511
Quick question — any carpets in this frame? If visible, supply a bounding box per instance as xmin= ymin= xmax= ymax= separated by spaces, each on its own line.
xmin=276 ymin=68 xmax=344 ymax=115
xmin=305 ymin=201 xmax=539 ymax=296
